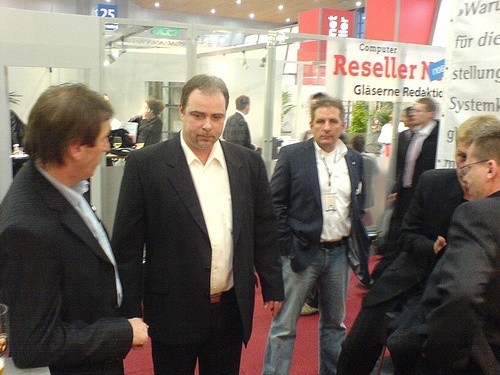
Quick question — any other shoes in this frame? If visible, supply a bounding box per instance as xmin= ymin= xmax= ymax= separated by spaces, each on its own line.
xmin=300 ymin=303 xmax=318 ymax=315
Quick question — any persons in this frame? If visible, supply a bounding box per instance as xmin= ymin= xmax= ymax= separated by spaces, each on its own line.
xmin=131 ymin=99 xmax=164 ymax=147
xmin=387 ymin=105 xmax=414 ymax=200
xmin=0 ymin=82 xmax=148 ymax=375
xmin=375 ymin=110 xmax=393 ymax=157
xmin=371 ymin=97 xmax=438 ymax=276
xmin=372 ymin=121 xmax=500 ymax=375
xmin=259 ymin=98 xmax=373 ymax=375
xmin=338 ymin=115 xmax=500 ymax=375
xmin=222 ymin=94 xmax=257 ymax=150
xmin=113 ymin=75 xmax=286 ymax=375
xmin=312 ymin=93 xmax=327 ymax=105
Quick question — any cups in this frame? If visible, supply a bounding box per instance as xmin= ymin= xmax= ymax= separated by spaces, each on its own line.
xmin=112 ymin=136 xmax=123 ymax=149
xmin=0 ymin=304 xmax=11 ymax=375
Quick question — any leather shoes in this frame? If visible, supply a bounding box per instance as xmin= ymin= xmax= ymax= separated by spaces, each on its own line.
xmin=363 ymin=278 xmax=374 ymax=287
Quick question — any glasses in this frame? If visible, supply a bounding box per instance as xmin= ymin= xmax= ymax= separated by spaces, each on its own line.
xmin=460 ymin=160 xmax=489 ymax=175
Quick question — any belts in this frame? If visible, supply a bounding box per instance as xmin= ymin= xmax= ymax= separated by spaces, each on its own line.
xmin=319 ymin=238 xmax=348 ymax=249
xmin=211 ymin=288 xmax=235 ymax=303
